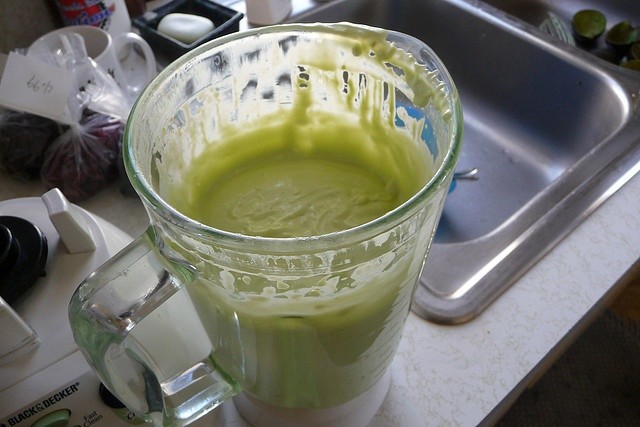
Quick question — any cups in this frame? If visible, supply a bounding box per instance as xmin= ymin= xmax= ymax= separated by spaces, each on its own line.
xmin=25 ymin=24 xmax=158 ymax=98
xmin=67 ymin=19 xmax=464 ymax=426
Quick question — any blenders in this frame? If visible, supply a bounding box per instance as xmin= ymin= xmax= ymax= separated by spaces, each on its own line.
xmin=0 ymin=188 xmax=213 ymax=427
